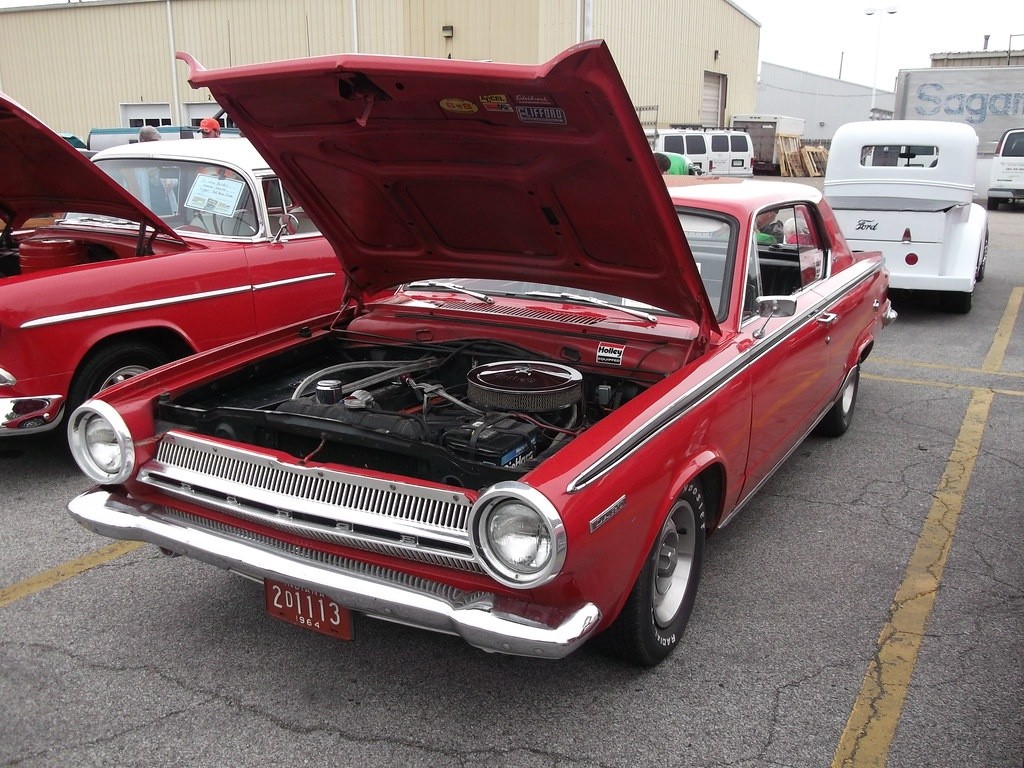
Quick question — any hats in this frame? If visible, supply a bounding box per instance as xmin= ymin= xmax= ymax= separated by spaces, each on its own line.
xmin=139 ymin=125 xmax=161 ymax=141
xmin=197 ymin=118 xmax=220 ymax=132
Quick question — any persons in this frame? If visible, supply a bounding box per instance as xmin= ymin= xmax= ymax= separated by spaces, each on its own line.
xmin=197 ymin=118 xmax=233 ymax=177
xmin=755 ymin=208 xmax=785 ymax=244
xmin=123 ymin=125 xmax=178 ymax=216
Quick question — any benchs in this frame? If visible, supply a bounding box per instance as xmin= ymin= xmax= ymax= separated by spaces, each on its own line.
xmin=688 ymin=249 xmax=800 ymax=306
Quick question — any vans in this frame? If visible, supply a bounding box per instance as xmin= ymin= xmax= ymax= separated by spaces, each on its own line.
xmin=642 ymin=126 xmax=756 ymax=178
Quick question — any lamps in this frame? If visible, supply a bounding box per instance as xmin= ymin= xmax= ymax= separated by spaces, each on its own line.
xmin=442 ymin=26 xmax=453 ymax=36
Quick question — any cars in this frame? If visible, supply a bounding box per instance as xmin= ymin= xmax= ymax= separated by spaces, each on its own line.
xmin=0 ymin=91 xmax=349 ymax=442
xmin=65 ymin=36 xmax=901 ymax=670
xmin=823 ymin=120 xmax=990 ymax=317
xmin=987 ymin=128 xmax=1024 ymax=211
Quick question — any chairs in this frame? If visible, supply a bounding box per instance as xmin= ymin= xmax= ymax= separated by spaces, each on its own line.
xmin=701 ymin=279 xmax=756 ymax=321
